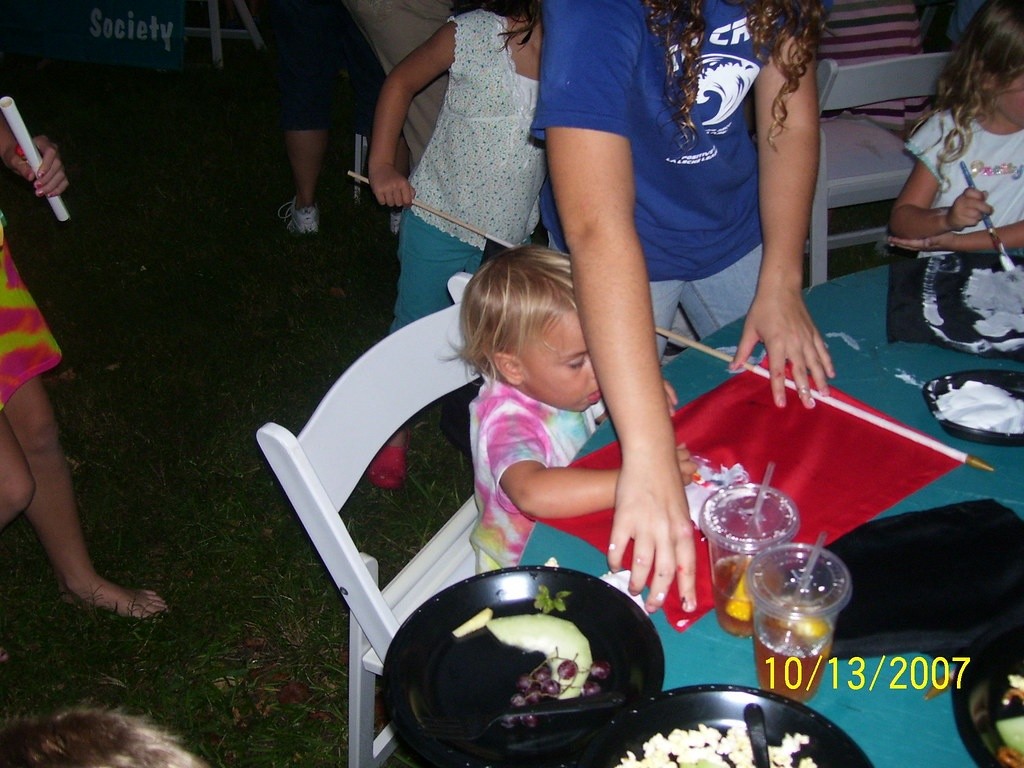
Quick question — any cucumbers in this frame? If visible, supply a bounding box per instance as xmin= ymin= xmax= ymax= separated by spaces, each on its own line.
xmin=996 ymin=715 xmax=1024 ymax=754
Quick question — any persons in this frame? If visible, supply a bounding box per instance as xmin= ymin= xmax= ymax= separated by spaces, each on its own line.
xmin=816 ymin=0 xmax=1024 ymax=263
xmin=0 ymin=96 xmax=170 ymax=619
xmin=0 ymin=705 xmax=210 ymax=768
xmin=276 ymin=0 xmax=835 ymax=611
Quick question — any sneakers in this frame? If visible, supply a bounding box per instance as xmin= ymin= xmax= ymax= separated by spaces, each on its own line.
xmin=278 ymin=195 xmax=319 ymax=233
xmin=390 ymin=212 xmax=402 ymax=233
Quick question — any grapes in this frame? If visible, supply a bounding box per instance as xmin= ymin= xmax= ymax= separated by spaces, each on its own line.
xmin=500 ymin=714 xmax=542 ymax=729
xmin=509 ymin=660 xmax=608 ymax=708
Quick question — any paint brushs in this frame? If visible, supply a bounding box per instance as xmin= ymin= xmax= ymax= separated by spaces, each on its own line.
xmin=692 ymin=473 xmax=712 ymax=487
xmin=959 ymin=160 xmax=1016 ymax=272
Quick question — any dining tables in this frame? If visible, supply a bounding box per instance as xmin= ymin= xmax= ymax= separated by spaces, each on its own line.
xmin=519 ymin=264 xmax=1024 ymax=768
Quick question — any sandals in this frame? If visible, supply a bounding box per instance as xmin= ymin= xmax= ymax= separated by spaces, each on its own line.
xmin=367 ymin=426 xmax=410 ymax=488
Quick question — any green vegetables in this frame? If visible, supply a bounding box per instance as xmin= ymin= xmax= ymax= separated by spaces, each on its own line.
xmin=533 ymin=585 xmax=572 ymax=614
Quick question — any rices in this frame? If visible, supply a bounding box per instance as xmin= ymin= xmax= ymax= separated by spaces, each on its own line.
xmin=615 ymin=732 xmax=822 ymax=768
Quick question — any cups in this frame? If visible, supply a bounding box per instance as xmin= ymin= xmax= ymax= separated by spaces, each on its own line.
xmin=700 ymin=485 xmax=802 ymax=637
xmin=747 ymin=545 xmax=853 ymax=702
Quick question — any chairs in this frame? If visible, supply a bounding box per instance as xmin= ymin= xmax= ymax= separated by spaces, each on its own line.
xmin=804 ymin=47 xmax=974 ymax=288
xmin=252 ymin=270 xmax=482 ymax=768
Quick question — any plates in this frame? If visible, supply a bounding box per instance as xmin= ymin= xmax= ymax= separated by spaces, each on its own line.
xmin=558 ymin=685 xmax=874 ymax=768
xmin=923 ymin=369 xmax=1024 ymax=446
xmin=954 ymin=623 xmax=1024 ymax=768
xmin=381 ymin=566 xmax=665 ymax=768
